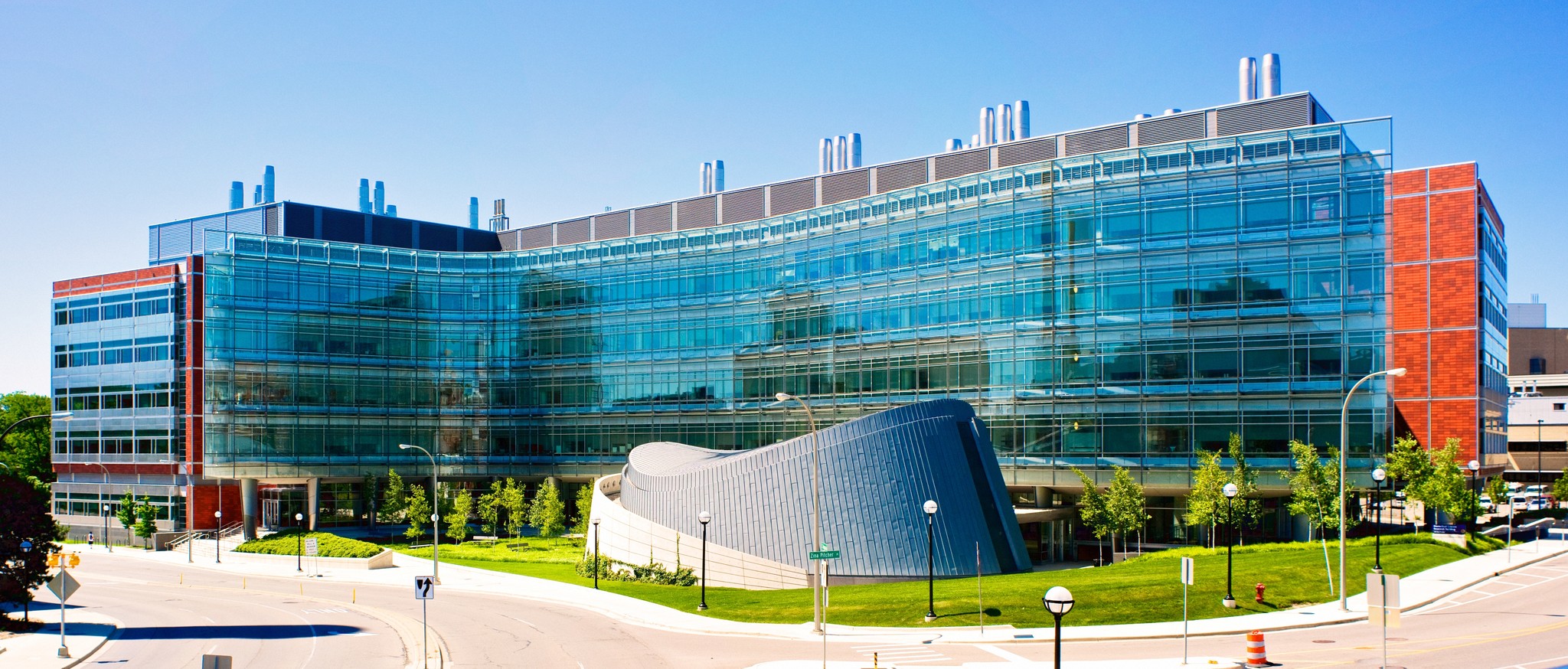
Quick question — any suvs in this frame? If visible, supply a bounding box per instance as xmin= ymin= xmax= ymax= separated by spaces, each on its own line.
xmin=1535 ymin=495 xmax=1561 ymax=509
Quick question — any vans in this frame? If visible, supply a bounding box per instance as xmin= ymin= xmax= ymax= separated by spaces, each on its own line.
xmin=1523 ymin=484 xmax=1549 ymax=497
xmin=1505 ymin=482 xmax=1526 ymax=497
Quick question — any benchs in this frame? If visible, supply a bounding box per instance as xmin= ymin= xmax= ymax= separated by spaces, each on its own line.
xmin=409 ymin=540 xmax=434 ymax=549
xmin=471 ymin=536 xmax=498 ymax=546
xmin=506 ymin=542 xmax=532 ymax=552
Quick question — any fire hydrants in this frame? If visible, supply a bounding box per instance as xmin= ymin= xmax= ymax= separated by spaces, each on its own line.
xmin=1254 ymin=582 xmax=1266 ymax=605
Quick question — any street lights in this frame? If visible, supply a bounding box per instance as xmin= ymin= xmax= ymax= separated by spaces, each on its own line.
xmin=1337 ymin=366 xmax=1409 ymax=611
xmin=1467 ymin=460 xmax=1481 ymax=542
xmin=398 ymin=442 xmax=441 ymax=586
xmin=1370 ymin=468 xmax=1387 ymax=570
xmin=83 ymin=462 xmax=113 ymax=552
xmin=775 ymin=391 xmax=824 ymax=634
xmin=697 ymin=509 xmax=715 ymax=605
xmin=1040 ymin=583 xmax=1077 ymax=669
xmin=590 ymin=516 xmax=601 ymax=589
xmin=1223 ymin=483 xmax=1240 ymax=599
xmin=214 ymin=510 xmax=222 ymax=564
xmin=101 ymin=504 xmax=110 ymax=548
xmin=922 ymin=498 xmax=939 ymax=618
xmin=159 ymin=459 xmax=193 ymax=562
xmin=296 ymin=512 xmax=302 ymax=571
xmin=1536 ymin=419 xmax=1544 ymax=510
xmin=20 ymin=541 xmax=33 ymax=630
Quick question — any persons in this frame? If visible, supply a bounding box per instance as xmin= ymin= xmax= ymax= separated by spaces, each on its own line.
xmin=87 ymin=532 xmax=94 ymax=550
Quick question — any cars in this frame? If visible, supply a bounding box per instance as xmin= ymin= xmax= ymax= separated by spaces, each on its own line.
xmin=1526 ymin=499 xmax=1552 ymax=512
xmin=1477 ymin=496 xmax=1498 ymax=513
xmin=1509 ymin=495 xmax=1532 ymax=511
